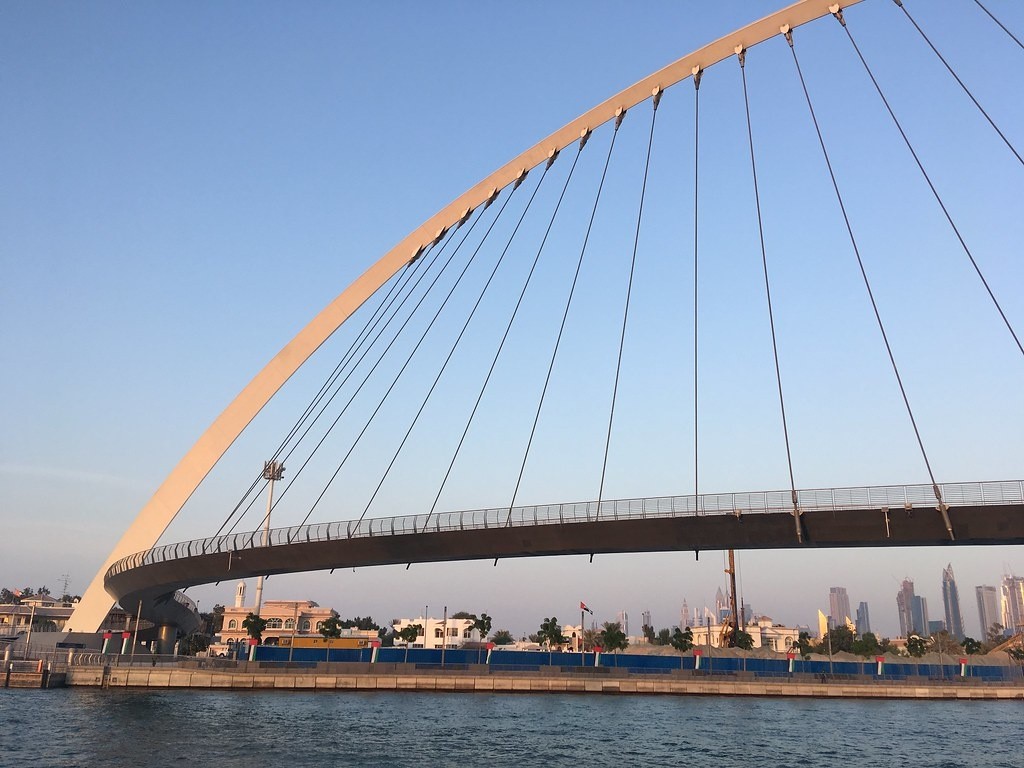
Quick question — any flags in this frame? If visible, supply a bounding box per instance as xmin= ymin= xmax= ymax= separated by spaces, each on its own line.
xmin=581 ymin=602 xmax=593 ymax=615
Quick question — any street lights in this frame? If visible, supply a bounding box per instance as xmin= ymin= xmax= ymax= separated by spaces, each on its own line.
xmin=289 ymin=603 xmax=298 ymax=662
xmin=827 ymin=616 xmax=833 ymax=673
xmin=442 ymin=606 xmax=447 ymax=667
xmin=937 ymin=629 xmax=948 ymax=678
